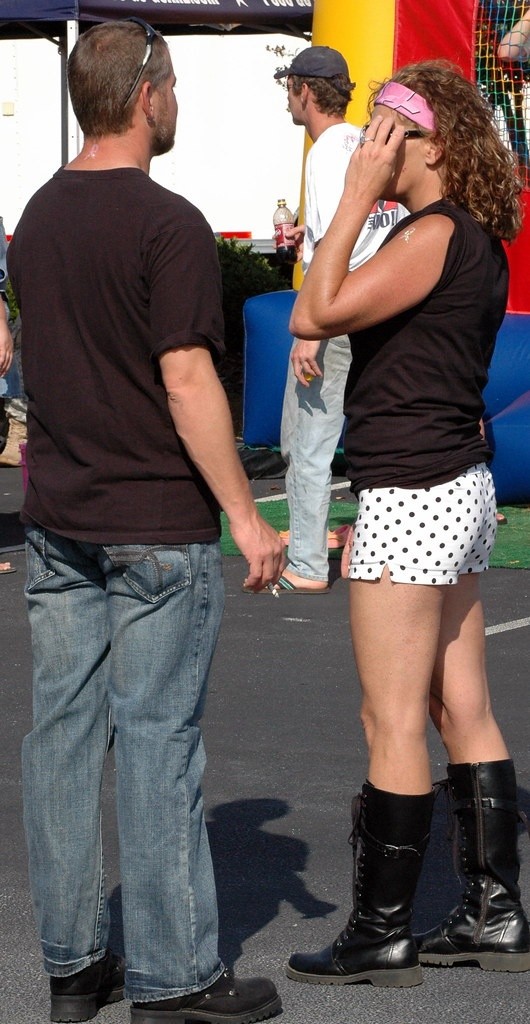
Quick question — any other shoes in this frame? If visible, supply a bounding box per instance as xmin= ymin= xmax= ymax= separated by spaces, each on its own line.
xmin=277 ymin=529 xmax=291 ymax=545
xmin=325 ymin=525 xmax=351 ymax=548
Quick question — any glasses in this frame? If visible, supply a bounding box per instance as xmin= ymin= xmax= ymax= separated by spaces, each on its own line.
xmin=121 ymin=17 xmax=159 ymax=114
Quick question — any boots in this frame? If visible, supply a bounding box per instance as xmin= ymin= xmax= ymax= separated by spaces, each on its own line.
xmin=284 ymin=778 xmax=435 ymax=987
xmin=407 ymin=759 xmax=530 ymax=972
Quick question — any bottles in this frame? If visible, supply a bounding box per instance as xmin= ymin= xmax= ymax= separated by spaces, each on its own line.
xmin=273 ymin=200 xmax=297 ymax=264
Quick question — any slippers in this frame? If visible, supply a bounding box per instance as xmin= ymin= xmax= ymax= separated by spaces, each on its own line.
xmin=241 ymin=576 xmax=330 ymax=595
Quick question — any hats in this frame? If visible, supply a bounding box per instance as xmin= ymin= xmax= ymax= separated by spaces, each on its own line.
xmin=274 ymin=46 xmax=351 ymax=83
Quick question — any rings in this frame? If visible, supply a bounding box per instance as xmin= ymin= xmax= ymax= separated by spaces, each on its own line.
xmin=360 ymin=136 xmax=374 ymax=148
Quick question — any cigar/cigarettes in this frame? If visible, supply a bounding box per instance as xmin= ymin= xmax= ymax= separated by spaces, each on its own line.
xmin=267 ymin=582 xmax=279 ymax=598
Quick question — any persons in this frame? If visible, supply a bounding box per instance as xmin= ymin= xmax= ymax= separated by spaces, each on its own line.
xmin=245 ymin=46 xmax=530 ymax=987
xmin=0 ymin=16 xmax=286 ymax=1024
xmin=498 ymin=8 xmax=530 ymax=62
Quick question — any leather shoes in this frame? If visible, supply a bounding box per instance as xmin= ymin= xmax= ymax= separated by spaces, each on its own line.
xmin=50 ymin=946 xmax=125 ymax=1021
xmin=129 ymin=966 xmax=282 ymax=1024
